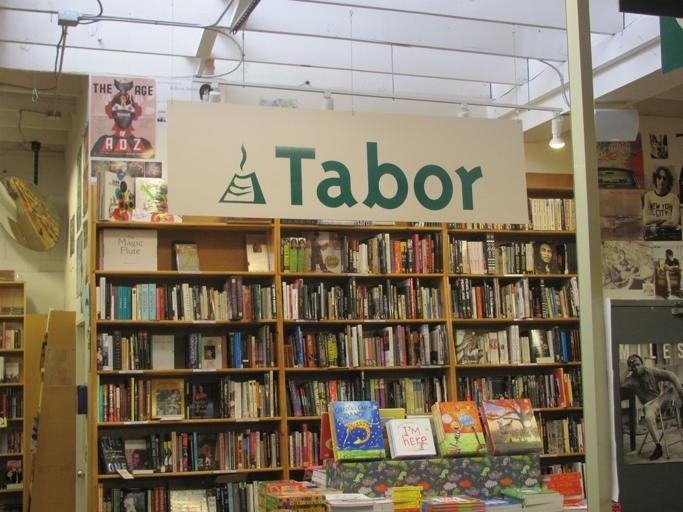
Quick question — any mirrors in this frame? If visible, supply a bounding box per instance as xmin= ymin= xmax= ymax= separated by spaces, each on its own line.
xmin=0 ymin=176 xmax=61 ymax=252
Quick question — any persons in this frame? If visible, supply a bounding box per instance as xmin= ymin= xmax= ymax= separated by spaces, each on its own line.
xmin=663 ymin=248 xmax=682 ymax=300
xmin=619 ymin=354 xmax=683 ymax=460
xmin=642 ymin=166 xmax=680 ymax=230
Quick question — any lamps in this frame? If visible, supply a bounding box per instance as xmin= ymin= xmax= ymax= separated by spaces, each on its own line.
xmin=549 ymin=112 xmax=566 ymax=149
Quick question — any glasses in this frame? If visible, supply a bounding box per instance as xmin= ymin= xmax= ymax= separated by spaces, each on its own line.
xmin=656 ymin=174 xmax=668 ymax=181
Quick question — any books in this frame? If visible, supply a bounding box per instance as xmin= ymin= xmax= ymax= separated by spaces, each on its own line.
xmin=95 ymin=276 xmax=277 ymax=320
xmin=280 ymin=230 xmax=443 ymax=274
xmin=95 ymin=171 xmax=169 ymax=223
xmin=454 ymin=324 xmax=581 ymax=365
xmin=98 ymin=228 xmax=157 ymax=271
xmin=528 ymin=198 xmax=577 ymax=232
xmin=99 ymin=371 xmax=283 ymax=422
xmin=447 ymin=235 xmax=576 ymax=276
xmin=259 ymin=400 xmax=564 ymax=512
xmin=281 ymin=276 xmax=442 ymax=321
xmin=97 ymin=324 xmax=275 ymax=371
xmin=457 ymin=368 xmax=585 ymax=408
xmin=285 ymin=371 xmax=447 ymax=416
xmin=543 ymin=461 xmax=588 ymax=506
xmin=99 ymin=426 xmax=280 ymax=474
xmin=173 ymin=243 xmax=200 ymax=272
xmin=452 ymin=277 xmax=580 ymax=319
xmin=98 ymin=481 xmax=258 ymax=512
xmin=284 ymin=322 xmax=449 ymax=367
xmin=534 ymin=413 xmax=586 ymax=455
xmin=243 ymin=231 xmax=271 ymax=273
xmin=225 ymin=217 xmax=533 ymax=232
xmin=0 ymin=305 xmax=24 ymax=490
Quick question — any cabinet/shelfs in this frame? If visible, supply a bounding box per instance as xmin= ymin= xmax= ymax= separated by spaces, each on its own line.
xmin=87 ymin=173 xmax=578 ymax=512
xmin=0 ymin=281 xmax=74 ymax=512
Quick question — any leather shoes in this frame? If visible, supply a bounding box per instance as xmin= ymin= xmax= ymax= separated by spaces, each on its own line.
xmin=650 ymin=444 xmax=663 ymax=461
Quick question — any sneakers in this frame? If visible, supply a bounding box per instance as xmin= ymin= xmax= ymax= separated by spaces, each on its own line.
xmin=666 ymin=291 xmax=671 ymax=300
